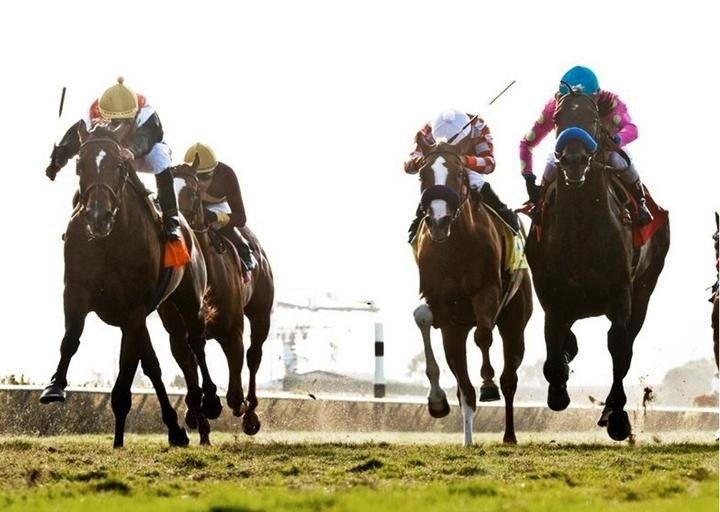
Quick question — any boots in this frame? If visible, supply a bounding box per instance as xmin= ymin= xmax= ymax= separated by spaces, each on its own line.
xmin=626 ymin=178 xmax=654 ymax=229
xmin=152 ymin=167 xmax=182 ymax=243
xmin=477 ymin=182 xmax=519 ymax=236
xmin=217 ymin=221 xmax=256 ymax=270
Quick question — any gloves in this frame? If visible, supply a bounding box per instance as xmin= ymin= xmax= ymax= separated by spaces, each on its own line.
xmin=525 ymin=174 xmax=543 ymax=204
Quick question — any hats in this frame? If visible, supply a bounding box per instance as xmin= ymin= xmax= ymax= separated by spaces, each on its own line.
xmin=432 ymin=109 xmax=471 ymax=146
xmin=560 ymin=65 xmax=601 ymax=97
xmin=98 ymin=77 xmax=139 ymax=120
xmin=183 ymin=142 xmax=219 ymax=174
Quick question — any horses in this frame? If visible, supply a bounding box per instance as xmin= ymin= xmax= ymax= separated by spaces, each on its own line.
xmin=412 ymin=130 xmax=534 ymax=448
xmin=37 ymin=118 xmax=223 ymax=448
xmin=523 ymin=87 xmax=671 ymax=442
xmin=156 ymin=151 xmax=275 ymax=447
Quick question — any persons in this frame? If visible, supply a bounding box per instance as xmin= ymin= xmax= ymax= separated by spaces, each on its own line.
xmin=519 ymin=64 xmax=654 ymax=230
xmin=44 ymin=77 xmax=183 ymax=242
xmin=403 ymin=105 xmax=520 ymax=245
xmin=173 ymin=141 xmax=259 ymax=272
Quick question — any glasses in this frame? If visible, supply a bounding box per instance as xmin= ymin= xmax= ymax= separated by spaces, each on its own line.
xmin=191 ymin=174 xmax=215 ymax=181
